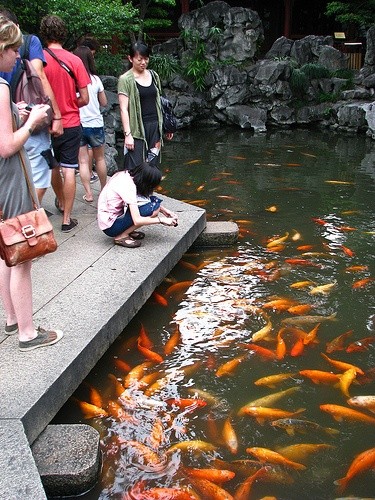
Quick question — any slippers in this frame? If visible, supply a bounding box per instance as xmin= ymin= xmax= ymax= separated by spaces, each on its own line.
xmin=114 ymin=235 xmax=140 ymax=248
xmin=130 ymin=232 xmax=145 ymax=239
xmin=83 ymin=194 xmax=93 ymax=204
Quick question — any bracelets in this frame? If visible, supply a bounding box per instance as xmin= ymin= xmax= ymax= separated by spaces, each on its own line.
xmin=158 ymin=216 xmax=162 ymax=224
xmin=125 ymin=131 xmax=132 ymax=136
xmin=26 ymin=123 xmax=33 ymax=134
xmin=51 ymin=117 xmax=62 ymax=120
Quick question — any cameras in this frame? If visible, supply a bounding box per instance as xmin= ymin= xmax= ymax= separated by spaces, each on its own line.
xmin=173 ymin=222 xmax=178 ymax=227
xmin=25 ymin=103 xmax=35 ymax=111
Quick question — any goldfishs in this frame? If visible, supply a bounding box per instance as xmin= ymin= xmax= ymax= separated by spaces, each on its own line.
xmin=77 ymin=150 xmax=375 ymax=500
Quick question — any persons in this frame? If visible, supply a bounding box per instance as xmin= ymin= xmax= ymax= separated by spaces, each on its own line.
xmin=0 ymin=15 xmax=108 ymax=234
xmin=98 ymin=161 xmax=177 ymax=248
xmin=0 ymin=15 xmax=66 ymax=352
xmin=118 ymin=41 xmax=174 ymax=170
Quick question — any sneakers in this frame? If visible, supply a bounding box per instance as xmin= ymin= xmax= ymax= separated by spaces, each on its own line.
xmin=5 ymin=323 xmax=18 ymax=334
xmin=19 ymin=326 xmax=64 ymax=351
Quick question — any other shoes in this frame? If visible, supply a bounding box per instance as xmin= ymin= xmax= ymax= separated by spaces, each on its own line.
xmin=55 ymin=197 xmax=64 ymax=213
xmin=61 ymin=218 xmax=78 ymax=232
xmin=90 ymin=175 xmax=98 ymax=183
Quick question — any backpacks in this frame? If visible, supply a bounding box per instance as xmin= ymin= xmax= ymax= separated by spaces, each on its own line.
xmin=11 ymin=36 xmax=55 ymax=132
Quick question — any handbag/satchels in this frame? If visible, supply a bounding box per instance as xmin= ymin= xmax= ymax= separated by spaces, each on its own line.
xmin=133 ymin=194 xmax=151 ymax=207
xmin=150 ymin=69 xmax=177 ymax=133
xmin=0 ymin=208 xmax=57 ymax=267
